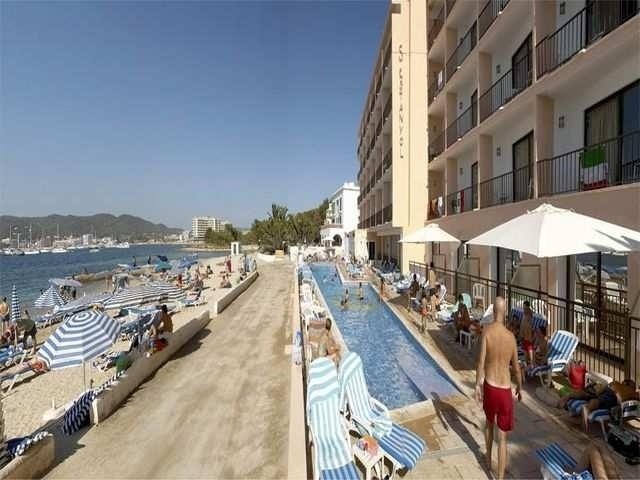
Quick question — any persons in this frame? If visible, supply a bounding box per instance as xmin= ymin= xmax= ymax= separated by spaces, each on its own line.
xmin=317 ymin=318 xmax=342 ymax=368
xmin=474 ymin=297 xmax=521 ymax=478
xmin=559 ymin=379 xmax=639 ymax=478
xmin=0 ymin=252 xmax=259 ymax=373
xmin=506 ymin=300 xmax=548 ymax=389
xmin=406 ymin=262 xmax=470 ymax=347
xmin=304 ymin=251 xmax=401 ymax=310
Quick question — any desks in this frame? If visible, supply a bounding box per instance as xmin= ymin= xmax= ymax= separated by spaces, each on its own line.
xmin=353 ymin=435 xmax=385 ymax=480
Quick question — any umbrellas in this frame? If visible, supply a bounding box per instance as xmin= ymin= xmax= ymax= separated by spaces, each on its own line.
xmin=396 ymin=222 xmax=460 ymax=264
xmin=0 ymin=431 xmax=48 ymax=469
xmin=465 ymin=203 xmax=640 ymax=305
xmin=60 ymin=372 xmax=123 ymax=438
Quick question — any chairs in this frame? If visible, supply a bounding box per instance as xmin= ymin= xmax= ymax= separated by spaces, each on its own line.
xmin=502 ymin=306 xmax=640 ymax=480
xmin=35 ymin=313 xmax=63 ymax=329
xmin=182 ymin=287 xmax=205 ymax=308
xmin=299 ymin=259 xmax=344 ymax=354
xmin=338 ymin=351 xmax=427 ymax=479
xmin=339 ymin=259 xmax=426 ymax=296
xmin=298 ymin=356 xmax=360 ymax=480
xmin=405 ymin=279 xmax=496 ymax=350
